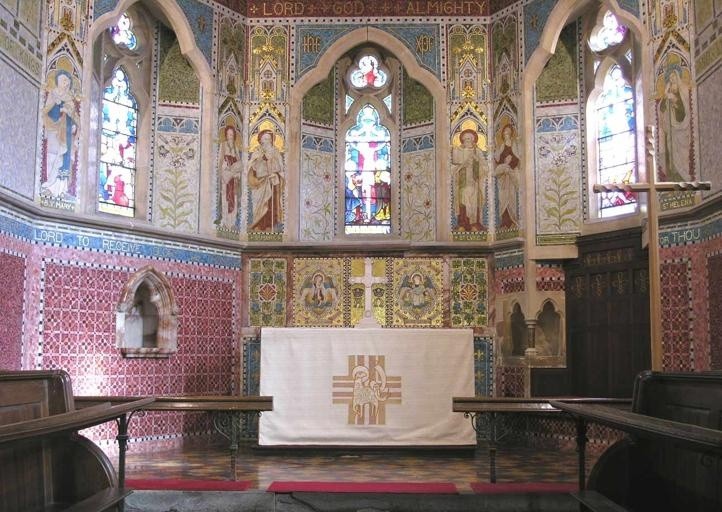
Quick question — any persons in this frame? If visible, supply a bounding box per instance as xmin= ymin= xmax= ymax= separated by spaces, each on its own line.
xmin=448 ymin=128 xmax=491 ymax=235
xmin=247 ymin=129 xmax=286 ymax=232
xmin=41 ymin=73 xmax=82 ymax=199
xmin=305 ymin=272 xmax=333 ymax=307
xmin=494 ymin=123 xmax=522 ymax=232
xmin=654 ymin=67 xmax=693 ymax=182
xmin=404 ymin=273 xmax=432 ymax=305
xmin=219 ymin=125 xmax=244 ymax=228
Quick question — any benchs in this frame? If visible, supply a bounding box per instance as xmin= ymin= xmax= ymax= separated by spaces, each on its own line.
xmin=0 ymin=369 xmax=133 ymax=512
xmin=569 ymin=371 xmax=722 ymax=511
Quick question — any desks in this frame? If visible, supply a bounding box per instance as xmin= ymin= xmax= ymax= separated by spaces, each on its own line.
xmin=453 ymin=396 xmax=633 ymax=483
xmin=1 ymin=397 xmax=156 ymax=512
xmin=72 ymin=394 xmax=274 ymax=480
xmin=547 ymin=400 xmax=722 ymax=511
